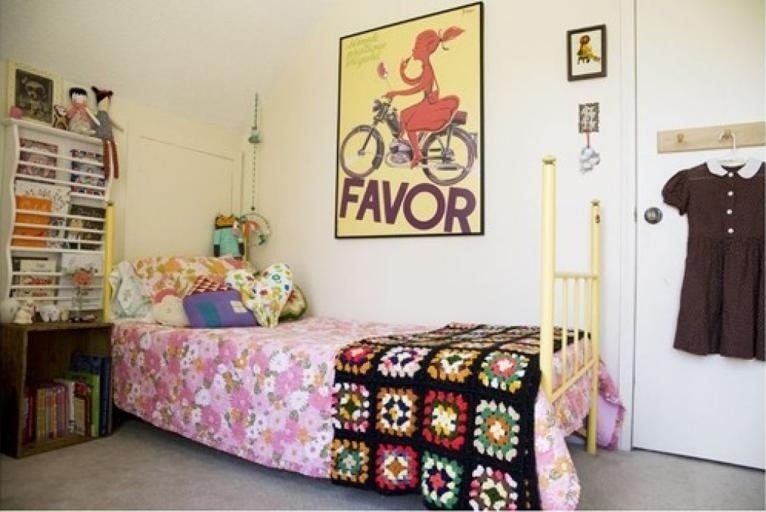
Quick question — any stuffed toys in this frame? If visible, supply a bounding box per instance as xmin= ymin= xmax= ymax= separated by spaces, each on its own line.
xmin=212 ymin=213 xmax=243 ymax=261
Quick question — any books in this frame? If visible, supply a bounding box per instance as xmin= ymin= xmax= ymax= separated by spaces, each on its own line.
xmin=65 ymin=372 xmax=100 ymax=437
xmin=76 ymin=400 xmax=90 ymax=437
xmin=70 ymin=380 xmax=75 ymax=433
xmin=70 ymin=355 xmax=112 ymax=436
xmin=37 ymin=382 xmax=69 ymax=442
xmin=21 ymin=394 xmax=35 ymax=444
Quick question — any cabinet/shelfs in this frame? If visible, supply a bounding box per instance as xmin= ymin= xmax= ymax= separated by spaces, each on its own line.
xmin=0 ymin=118 xmax=119 ymax=459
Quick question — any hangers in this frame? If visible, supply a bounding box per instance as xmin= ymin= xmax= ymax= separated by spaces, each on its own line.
xmin=687 ymin=130 xmax=766 ymax=181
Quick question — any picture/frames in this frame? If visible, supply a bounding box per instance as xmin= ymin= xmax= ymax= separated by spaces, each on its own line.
xmin=335 ymin=1 xmax=485 ymax=239
xmin=566 ymin=24 xmax=608 ymax=82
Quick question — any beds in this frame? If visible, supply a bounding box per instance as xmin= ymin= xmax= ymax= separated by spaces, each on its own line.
xmin=103 ymin=156 xmax=600 ymax=510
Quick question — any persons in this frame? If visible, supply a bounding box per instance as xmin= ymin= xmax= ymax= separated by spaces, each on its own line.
xmin=92 ymin=87 xmax=124 ymax=179
xmin=66 ymin=88 xmax=100 ymax=134
